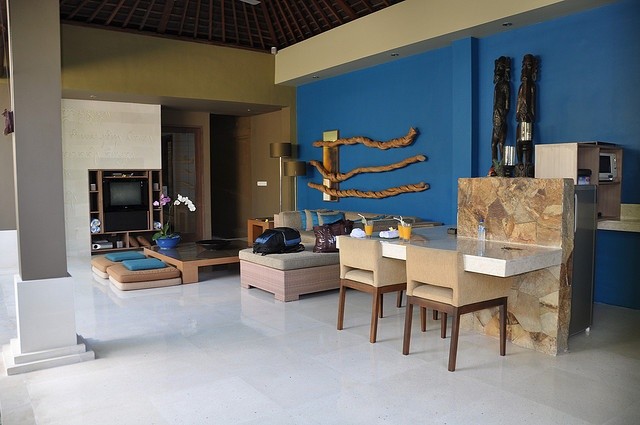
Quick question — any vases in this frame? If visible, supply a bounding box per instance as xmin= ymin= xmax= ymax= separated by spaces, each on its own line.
xmin=155 ymin=235 xmax=180 ymax=249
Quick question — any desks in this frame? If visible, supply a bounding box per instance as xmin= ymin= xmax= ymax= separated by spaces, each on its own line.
xmin=247 ymin=219 xmax=274 ymax=248
xmin=144 ymin=240 xmax=252 ymax=284
xmin=336 ymin=224 xmax=561 ymax=277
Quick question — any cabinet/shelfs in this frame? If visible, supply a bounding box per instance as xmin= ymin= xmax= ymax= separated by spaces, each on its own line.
xmin=535 ymin=142 xmax=623 ymax=222
xmin=88 ymin=169 xmax=164 ymax=252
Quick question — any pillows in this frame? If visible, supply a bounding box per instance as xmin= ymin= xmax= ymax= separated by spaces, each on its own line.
xmin=317 ymin=210 xmax=340 ymax=226
xmin=320 ymin=211 xmax=344 ymax=225
xmin=121 ymin=257 xmax=166 ymax=271
xmin=353 ymin=214 xmax=385 ymax=222
xmin=299 ymin=211 xmax=306 ymax=231
xmin=129 ymin=236 xmax=140 ymax=247
xmin=104 ymin=249 xmax=146 ymax=262
xmin=303 ymin=209 xmax=327 ymax=232
xmin=136 ymin=235 xmax=151 ymax=246
xmin=309 ymin=211 xmax=319 ymax=229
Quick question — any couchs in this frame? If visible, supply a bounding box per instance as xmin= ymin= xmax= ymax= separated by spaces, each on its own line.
xmin=239 ymin=209 xmax=421 ymax=302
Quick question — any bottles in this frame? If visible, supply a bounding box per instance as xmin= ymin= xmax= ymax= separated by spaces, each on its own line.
xmin=478 ymin=219 xmax=485 ymax=241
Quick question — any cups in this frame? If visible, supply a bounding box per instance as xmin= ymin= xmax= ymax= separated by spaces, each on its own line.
xmin=366 ymin=225 xmax=373 ymax=240
xmin=364 ymin=221 xmax=374 ymax=235
xmin=403 ymin=223 xmax=412 ymax=243
xmin=398 ymin=224 xmax=404 ymax=240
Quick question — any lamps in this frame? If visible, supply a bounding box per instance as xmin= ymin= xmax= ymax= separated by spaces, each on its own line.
xmin=270 ymin=143 xmax=291 ymax=212
xmin=284 ymin=161 xmax=306 ymax=211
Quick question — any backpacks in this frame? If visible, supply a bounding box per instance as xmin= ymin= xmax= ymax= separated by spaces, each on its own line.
xmin=252 ymin=226 xmax=305 ymax=257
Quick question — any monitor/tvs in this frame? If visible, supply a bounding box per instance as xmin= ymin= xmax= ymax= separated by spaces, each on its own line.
xmin=102 ymin=178 xmax=149 ymax=213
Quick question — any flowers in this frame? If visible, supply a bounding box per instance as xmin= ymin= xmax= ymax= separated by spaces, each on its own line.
xmin=152 ymin=193 xmax=197 ymax=241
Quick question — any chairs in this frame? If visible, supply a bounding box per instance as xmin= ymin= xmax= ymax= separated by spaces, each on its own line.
xmin=337 ymin=235 xmax=407 ymax=342
xmin=402 ymin=243 xmax=512 ymax=372
